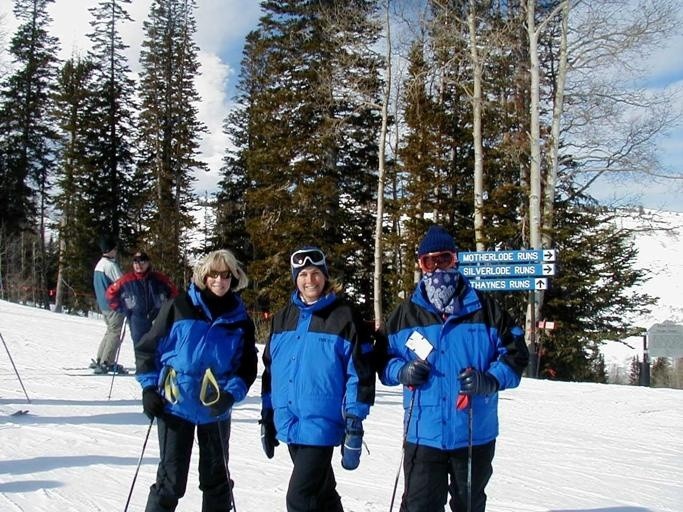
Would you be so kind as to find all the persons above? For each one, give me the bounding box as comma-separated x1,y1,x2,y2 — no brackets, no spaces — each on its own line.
372,225,528,510
260,245,376,510
107,251,179,361
91,236,129,373
133,249,258,510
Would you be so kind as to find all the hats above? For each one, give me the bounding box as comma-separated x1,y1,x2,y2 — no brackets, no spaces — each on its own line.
131,250,150,261
98,236,117,254
417,223,455,255
291,246,330,286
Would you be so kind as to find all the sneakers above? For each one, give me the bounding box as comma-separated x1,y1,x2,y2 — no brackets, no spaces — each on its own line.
92,359,102,367
98,363,124,373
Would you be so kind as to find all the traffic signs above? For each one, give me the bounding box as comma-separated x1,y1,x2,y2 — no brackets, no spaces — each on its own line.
464,277,549,291
457,263,555,278
457,248,556,263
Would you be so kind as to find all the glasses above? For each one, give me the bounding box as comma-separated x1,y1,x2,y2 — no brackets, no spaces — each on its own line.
206,270,233,279
420,250,455,273
133,261,149,265
291,249,326,268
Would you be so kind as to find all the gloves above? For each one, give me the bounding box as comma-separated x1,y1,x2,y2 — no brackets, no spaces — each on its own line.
143,385,166,419
398,357,433,387
117,306,132,319
203,391,235,416
456,367,498,395
259,410,280,459
338,430,363,471
